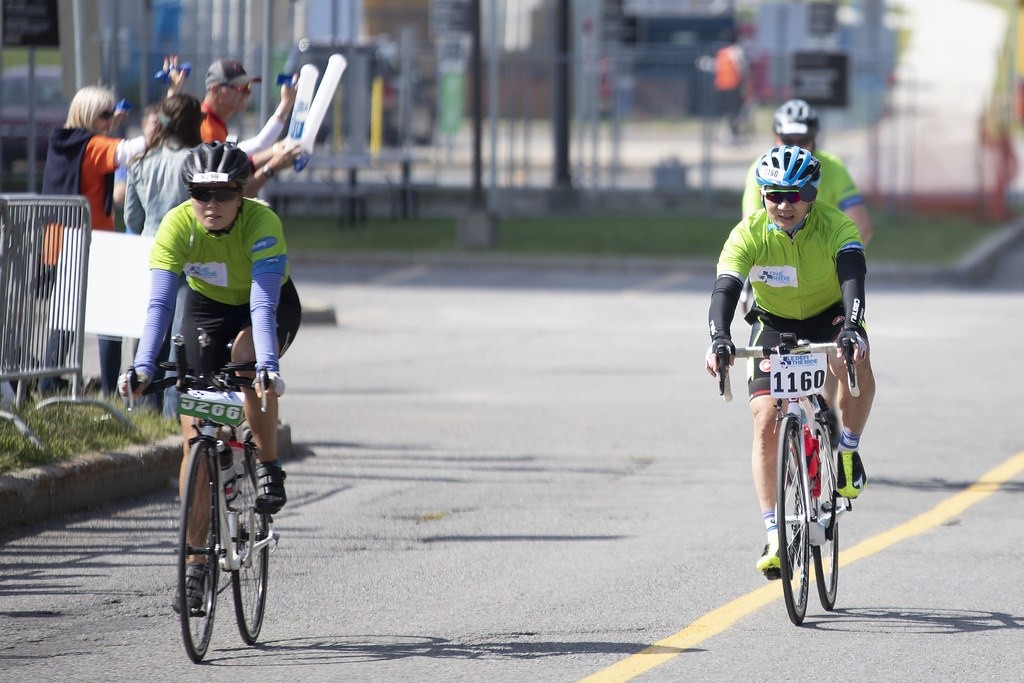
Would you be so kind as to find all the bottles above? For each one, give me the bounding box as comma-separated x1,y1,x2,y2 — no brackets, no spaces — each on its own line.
790,425,822,498
228,436,245,509
217,439,242,502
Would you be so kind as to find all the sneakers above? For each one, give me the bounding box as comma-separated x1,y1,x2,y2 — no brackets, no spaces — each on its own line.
254,467,288,514
836,447,869,498
173,563,210,613
757,542,792,579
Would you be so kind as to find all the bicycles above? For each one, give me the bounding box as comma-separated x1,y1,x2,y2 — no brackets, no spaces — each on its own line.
714,330,848,626
121,335,275,665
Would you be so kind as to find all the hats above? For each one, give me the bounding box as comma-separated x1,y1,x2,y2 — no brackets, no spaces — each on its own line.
205,57,262,90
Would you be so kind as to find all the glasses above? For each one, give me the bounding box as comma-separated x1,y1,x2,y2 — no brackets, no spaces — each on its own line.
760,187,801,204
100,111,114,120
776,132,814,148
221,82,252,96
187,185,243,202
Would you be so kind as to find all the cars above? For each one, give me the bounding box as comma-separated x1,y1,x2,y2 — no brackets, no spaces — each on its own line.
0,70,68,172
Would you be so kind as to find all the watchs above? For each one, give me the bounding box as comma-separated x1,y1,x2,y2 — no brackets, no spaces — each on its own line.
262,162,273,178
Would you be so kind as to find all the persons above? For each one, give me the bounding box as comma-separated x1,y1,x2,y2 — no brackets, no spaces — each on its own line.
230,136,301,198
35,55,186,402
122,93,216,412
741,99,874,250
116,142,303,616
586,54,616,121
714,22,769,147
199,59,299,155
705,146,875,580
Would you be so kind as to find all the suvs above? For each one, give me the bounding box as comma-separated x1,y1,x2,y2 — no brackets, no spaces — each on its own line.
281,42,407,149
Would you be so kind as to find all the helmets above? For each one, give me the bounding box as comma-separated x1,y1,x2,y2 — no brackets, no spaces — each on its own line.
181,140,250,189
773,99,821,136
756,146,822,191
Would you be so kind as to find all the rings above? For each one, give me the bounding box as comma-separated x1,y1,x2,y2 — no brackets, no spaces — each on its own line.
169,64,175,70
